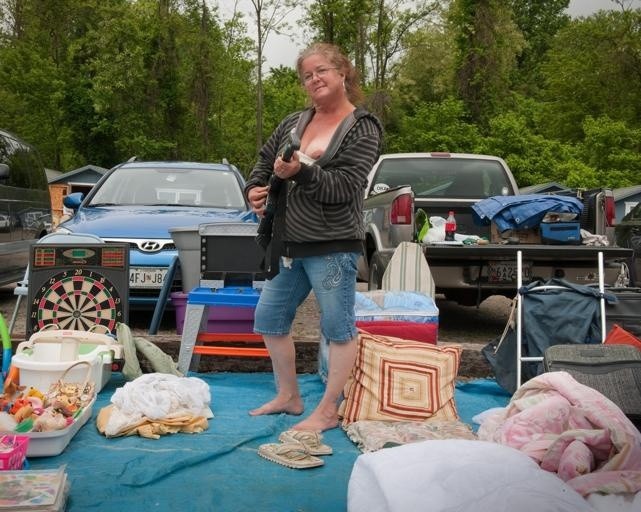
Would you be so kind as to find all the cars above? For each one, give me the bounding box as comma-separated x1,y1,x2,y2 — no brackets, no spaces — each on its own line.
30,156,261,306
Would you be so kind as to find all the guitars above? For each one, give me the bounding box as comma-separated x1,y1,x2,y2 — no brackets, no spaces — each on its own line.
255,127,301,251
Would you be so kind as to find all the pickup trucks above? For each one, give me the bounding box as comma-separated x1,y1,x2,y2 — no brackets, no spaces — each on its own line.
357,153,633,305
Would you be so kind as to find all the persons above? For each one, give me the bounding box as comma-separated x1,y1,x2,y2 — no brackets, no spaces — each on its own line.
243,43,383,433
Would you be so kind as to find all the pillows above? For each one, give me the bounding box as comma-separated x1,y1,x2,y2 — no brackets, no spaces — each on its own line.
334,325,480,455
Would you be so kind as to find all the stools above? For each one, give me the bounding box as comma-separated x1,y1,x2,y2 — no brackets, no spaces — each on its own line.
176,285,270,374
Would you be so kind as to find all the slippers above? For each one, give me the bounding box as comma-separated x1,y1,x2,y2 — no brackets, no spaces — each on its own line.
257,430,332,469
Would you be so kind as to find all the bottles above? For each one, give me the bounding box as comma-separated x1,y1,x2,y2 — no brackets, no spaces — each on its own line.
445,211,457,241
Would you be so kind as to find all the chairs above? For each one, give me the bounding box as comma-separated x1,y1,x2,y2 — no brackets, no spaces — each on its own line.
134,183,228,206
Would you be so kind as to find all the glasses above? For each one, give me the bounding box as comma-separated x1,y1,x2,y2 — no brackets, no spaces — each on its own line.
303,65,339,82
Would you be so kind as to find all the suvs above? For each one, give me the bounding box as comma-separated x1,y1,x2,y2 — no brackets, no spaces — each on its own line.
0,127,55,287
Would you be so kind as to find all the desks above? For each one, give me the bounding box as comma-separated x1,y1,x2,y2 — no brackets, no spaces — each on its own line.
411,245,634,394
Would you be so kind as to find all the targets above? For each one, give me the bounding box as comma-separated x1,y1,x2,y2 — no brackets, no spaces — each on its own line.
30,270,122,336
